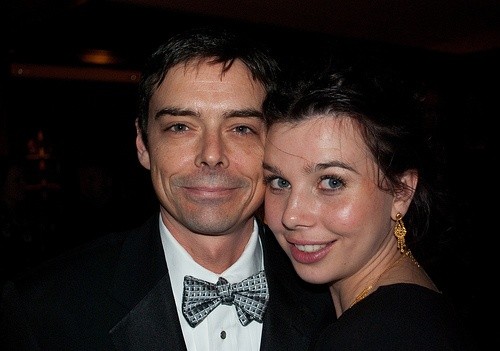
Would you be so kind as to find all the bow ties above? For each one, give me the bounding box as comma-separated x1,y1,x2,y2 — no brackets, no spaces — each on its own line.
180,270,270,328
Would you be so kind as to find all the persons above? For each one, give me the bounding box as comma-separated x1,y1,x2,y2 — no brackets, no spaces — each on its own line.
257,62,475,351
19,24,338,351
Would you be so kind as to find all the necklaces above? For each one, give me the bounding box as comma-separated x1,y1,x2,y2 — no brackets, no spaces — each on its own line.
346,249,413,312
